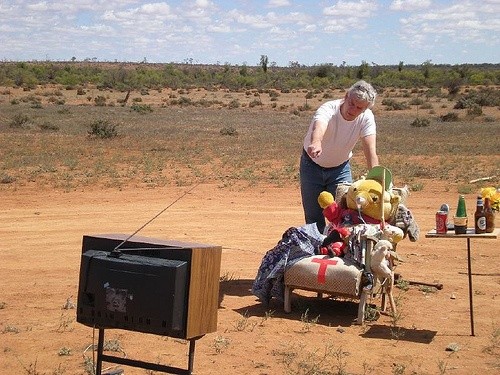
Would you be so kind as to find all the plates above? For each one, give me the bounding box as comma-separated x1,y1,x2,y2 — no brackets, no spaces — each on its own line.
447,223,453,229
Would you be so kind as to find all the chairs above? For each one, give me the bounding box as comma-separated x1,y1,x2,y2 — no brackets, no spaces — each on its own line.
280,183,406,328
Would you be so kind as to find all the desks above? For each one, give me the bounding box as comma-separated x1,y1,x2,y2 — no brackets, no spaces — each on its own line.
426,224,500,336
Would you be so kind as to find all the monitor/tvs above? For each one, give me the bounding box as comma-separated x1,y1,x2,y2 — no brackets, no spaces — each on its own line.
76,234,222,338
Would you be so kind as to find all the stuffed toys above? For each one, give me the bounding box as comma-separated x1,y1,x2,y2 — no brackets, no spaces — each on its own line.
314,164,402,258
370,239,393,293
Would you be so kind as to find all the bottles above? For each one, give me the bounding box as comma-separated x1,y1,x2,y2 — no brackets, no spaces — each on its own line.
454,194,467,234
482,197,494,234
475,194,487,234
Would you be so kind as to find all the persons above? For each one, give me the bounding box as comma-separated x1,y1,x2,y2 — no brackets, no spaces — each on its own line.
300,80,378,235
